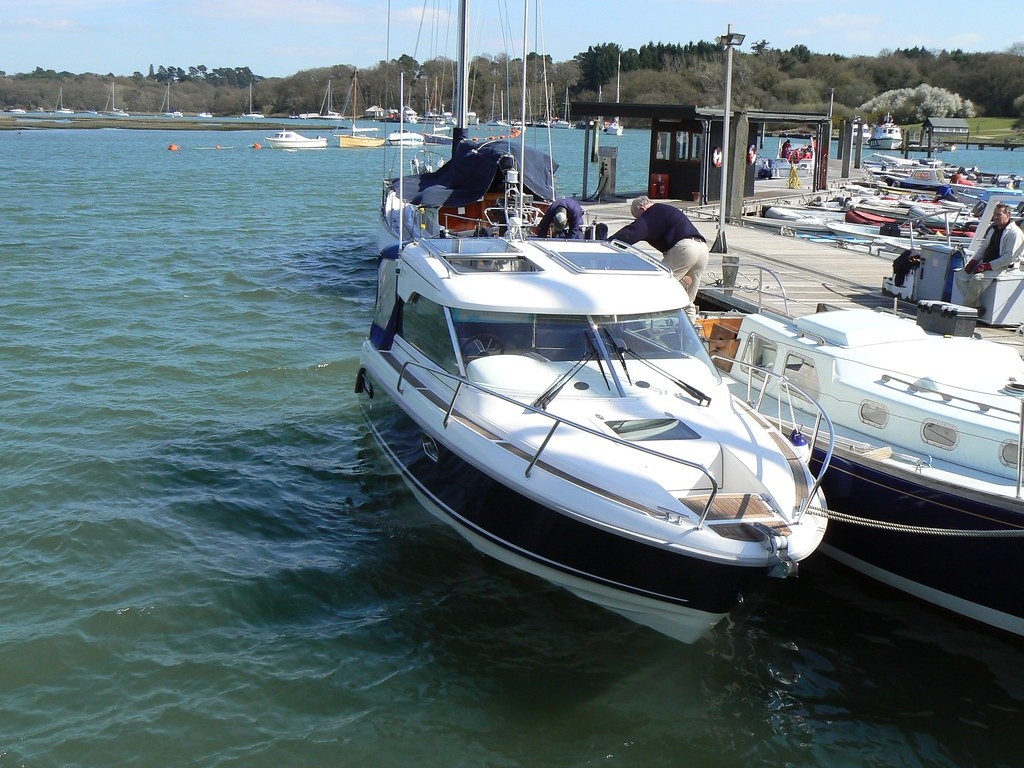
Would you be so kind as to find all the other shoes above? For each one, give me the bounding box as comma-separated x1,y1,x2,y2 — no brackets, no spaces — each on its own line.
972,306,986,317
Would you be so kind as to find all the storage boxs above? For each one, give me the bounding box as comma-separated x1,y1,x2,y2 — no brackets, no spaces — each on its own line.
916,300,980,339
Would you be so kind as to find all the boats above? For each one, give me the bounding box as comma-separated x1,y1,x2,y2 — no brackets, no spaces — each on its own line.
867,112,904,151
196,112,213,118
674,254,1023,533
906,138,954,152
851,113,872,146
265,128,329,148
387,126,426,147
760,149,1023,268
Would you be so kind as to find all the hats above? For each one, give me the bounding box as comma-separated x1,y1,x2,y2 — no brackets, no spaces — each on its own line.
553,207,567,228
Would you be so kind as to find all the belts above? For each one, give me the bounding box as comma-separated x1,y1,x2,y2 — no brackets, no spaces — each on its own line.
685,236,705,242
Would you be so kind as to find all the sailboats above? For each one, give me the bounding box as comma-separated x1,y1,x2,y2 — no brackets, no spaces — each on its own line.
421,70,455,145
351,1,837,646
52,84,75,114
235,82,265,120
374,53,624,136
95,80,130,117
293,78,345,121
330,65,387,147
155,80,185,119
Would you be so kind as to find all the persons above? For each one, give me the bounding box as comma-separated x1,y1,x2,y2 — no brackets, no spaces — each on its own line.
955,204,1024,317
781,139,792,157
534,199,586,240
610,195,710,302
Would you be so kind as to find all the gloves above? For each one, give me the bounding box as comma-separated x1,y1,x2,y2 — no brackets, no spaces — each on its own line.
965,259,979,274
974,262,991,274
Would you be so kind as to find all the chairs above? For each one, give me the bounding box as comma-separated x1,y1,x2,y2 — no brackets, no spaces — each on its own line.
583,338,628,359
452,336,486,357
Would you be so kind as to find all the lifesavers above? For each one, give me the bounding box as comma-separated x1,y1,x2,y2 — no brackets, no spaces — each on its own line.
748,145,757,165
713,148,723,167
